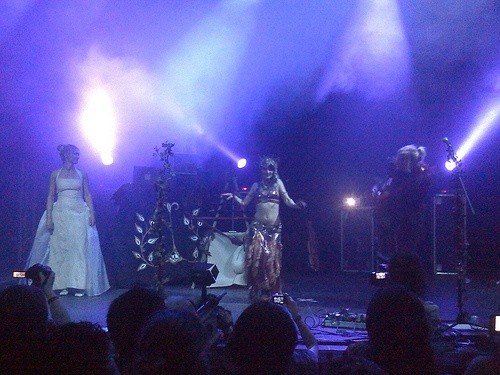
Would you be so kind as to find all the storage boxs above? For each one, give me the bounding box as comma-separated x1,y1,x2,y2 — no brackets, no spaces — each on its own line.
433,194,466,275
342,208,377,273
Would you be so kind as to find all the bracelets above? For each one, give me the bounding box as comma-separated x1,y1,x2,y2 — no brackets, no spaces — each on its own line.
293,311,299,317
233,193,234,199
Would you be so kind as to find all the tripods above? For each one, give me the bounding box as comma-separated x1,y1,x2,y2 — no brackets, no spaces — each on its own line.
432,172,489,336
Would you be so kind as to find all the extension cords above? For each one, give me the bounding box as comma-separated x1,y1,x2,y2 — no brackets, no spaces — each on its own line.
324,319,367,330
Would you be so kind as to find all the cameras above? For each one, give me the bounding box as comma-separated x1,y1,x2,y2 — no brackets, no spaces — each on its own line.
489,313,500,335
12,263,51,281
371,271,390,284
272,292,286,305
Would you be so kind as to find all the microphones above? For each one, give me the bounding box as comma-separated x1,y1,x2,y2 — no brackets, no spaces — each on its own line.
442,137,453,151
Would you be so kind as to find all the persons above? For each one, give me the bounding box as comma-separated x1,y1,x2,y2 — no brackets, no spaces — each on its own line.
0,255,500,375
22,144,110,297
220,158,306,304
375,145,433,270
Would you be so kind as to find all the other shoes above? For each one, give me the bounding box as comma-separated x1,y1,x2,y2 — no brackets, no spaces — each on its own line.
60,289,69,295
75,290,85,296
